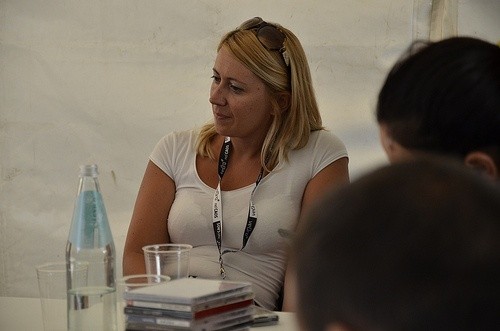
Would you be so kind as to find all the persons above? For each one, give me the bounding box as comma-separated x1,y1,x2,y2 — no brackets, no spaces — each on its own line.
282,155,500,331
120,15,354,315
373,32,500,177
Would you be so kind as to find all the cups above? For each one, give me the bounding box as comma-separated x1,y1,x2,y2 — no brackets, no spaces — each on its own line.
37,260,91,331
118,271,170,298
142,243,193,287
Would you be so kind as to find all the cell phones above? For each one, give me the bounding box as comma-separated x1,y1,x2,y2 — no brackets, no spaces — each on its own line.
250,304,278,328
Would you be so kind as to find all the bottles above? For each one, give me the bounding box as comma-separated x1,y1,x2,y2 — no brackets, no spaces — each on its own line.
64,163,121,330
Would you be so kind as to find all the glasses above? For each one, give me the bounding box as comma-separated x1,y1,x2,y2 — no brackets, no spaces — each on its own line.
242,17,290,71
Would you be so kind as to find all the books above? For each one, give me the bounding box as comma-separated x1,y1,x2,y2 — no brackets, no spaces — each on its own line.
122,275,258,331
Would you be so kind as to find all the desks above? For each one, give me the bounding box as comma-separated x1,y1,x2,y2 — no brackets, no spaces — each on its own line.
0,296,309,331
